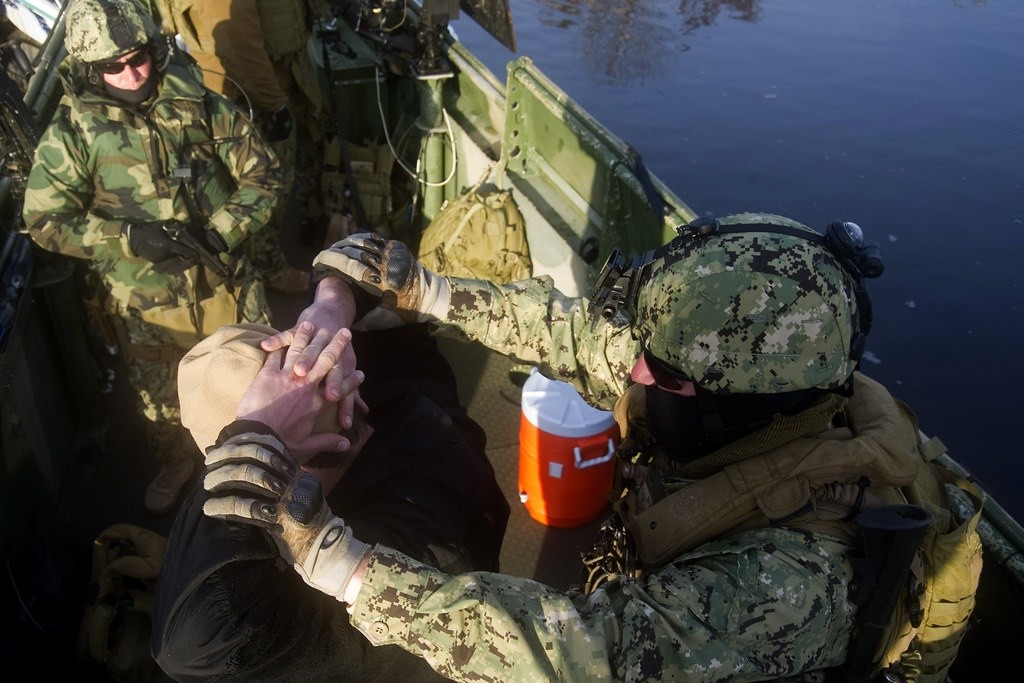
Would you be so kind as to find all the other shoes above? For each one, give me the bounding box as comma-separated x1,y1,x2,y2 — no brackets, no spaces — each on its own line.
269,266,311,295
146,460,194,513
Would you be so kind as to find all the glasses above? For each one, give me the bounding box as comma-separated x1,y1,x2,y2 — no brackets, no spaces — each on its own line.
94,46,152,75
640,344,691,391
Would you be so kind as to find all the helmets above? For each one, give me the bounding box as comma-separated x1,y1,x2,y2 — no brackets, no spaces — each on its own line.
64,0,157,61
629,210,863,395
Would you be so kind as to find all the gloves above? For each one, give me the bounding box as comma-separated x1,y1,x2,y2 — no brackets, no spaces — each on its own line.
312,233,447,334
266,111,293,142
202,432,372,601
128,220,228,273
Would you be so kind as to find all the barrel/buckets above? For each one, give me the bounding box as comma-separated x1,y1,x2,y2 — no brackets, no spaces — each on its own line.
518,365,619,528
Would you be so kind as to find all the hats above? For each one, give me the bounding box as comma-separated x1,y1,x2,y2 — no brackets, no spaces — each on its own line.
178,321,344,466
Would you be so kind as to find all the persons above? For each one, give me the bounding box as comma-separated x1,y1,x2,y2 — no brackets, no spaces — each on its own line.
0,0,885,682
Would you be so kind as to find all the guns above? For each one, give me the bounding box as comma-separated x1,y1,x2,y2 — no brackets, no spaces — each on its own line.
165,220,228,278
